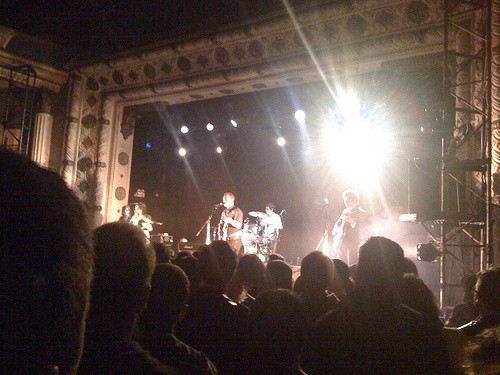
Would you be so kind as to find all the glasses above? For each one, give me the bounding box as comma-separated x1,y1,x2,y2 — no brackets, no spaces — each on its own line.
470,285,490,298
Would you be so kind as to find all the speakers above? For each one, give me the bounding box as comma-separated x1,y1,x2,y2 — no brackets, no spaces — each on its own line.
409,155,466,215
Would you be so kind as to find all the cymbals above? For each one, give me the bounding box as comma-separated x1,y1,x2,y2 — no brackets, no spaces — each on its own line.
248,211,267,218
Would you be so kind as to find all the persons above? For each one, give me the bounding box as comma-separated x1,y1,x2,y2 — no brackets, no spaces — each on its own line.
0,192,500,375
0,146,92,375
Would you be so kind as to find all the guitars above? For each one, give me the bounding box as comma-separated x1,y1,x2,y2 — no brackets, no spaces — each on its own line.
218,206,237,241
333,207,360,250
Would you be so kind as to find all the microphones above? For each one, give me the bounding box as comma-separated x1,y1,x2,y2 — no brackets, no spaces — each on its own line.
215,203,222,209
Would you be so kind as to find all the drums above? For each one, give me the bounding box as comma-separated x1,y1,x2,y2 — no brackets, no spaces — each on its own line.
253,237,271,254
